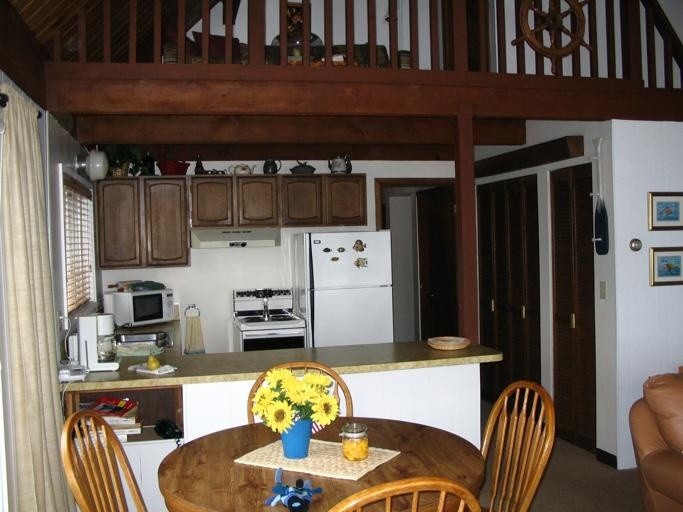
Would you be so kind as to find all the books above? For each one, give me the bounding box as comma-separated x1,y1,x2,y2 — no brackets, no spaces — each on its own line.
73,396,142,449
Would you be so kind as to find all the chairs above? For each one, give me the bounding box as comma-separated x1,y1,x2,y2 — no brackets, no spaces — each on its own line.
247,362,352,424
61,409,148,512
328,477,482,512
482,380,556,512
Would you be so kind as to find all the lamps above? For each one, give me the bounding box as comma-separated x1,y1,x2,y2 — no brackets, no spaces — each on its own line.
75,150,109,181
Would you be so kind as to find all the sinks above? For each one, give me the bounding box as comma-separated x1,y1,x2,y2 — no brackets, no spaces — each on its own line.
115,329,174,348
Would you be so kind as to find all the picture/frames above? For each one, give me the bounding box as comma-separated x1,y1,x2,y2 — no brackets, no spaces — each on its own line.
647,191,683,230
649,246,683,286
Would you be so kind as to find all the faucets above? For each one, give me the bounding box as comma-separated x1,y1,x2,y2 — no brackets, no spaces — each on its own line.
117,321,135,332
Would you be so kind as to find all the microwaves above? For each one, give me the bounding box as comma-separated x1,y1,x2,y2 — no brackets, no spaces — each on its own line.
105,289,175,328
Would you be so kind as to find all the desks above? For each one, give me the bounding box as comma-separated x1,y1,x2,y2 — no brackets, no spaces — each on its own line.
158,417,486,512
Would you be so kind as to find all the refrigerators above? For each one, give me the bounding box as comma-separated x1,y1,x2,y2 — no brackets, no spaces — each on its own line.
291,230,394,349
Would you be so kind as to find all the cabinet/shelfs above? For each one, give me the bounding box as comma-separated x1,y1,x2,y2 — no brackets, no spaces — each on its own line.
191,174,279,228
280,173,367,227
97,178,187,267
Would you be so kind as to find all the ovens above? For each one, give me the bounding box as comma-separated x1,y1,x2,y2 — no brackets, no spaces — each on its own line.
242,331,308,350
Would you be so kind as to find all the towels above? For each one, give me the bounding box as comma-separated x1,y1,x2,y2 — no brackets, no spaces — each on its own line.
182,317,205,357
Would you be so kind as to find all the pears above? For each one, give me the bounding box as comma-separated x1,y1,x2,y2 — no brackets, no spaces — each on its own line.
145,356,161,371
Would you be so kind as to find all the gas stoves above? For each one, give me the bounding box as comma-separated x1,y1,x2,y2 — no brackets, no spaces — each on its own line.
233,308,306,331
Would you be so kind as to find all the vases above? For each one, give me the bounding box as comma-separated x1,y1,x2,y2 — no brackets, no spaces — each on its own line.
281,420,312,459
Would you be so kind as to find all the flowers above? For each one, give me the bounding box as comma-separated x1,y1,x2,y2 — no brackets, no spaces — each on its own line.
251,367,341,434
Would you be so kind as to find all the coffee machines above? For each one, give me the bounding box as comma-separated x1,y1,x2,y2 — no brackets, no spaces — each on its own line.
69,315,121,371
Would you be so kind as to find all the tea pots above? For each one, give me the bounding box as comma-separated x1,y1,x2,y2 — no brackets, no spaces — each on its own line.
263,157,282,174
229,163,256,175
328,153,352,174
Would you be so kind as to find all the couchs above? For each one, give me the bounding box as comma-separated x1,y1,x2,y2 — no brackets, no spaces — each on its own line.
629,366,683,512
